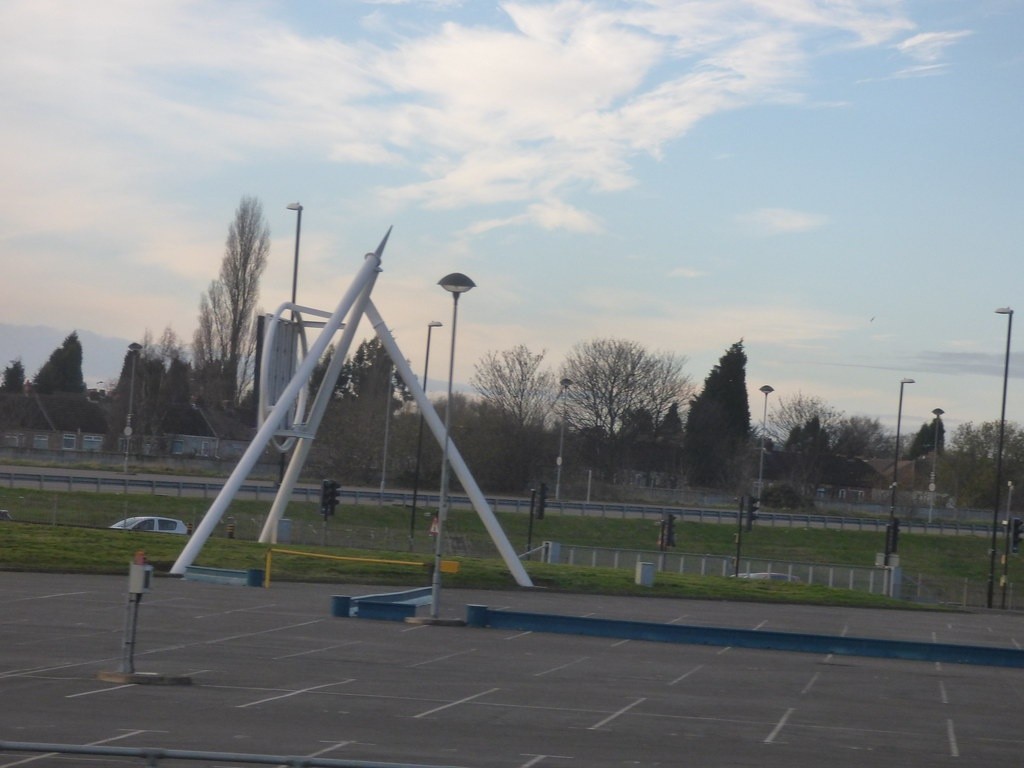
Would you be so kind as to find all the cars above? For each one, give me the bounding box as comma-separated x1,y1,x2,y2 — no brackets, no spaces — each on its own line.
110,516,188,535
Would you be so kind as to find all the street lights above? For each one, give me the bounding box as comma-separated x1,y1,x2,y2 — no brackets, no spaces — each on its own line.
124,340,143,475
986,305,1015,609
753,384,775,519
407,321,443,539
883,378,917,562
427,272,476,616
286,201,305,321
926,408,945,523
554,378,574,498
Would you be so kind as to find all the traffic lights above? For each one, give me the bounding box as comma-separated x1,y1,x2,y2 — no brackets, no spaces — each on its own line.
747,496,759,533
322,480,333,514
1009,516,1023,553
331,480,343,507
534,483,548,521
664,514,677,547
889,518,901,552
